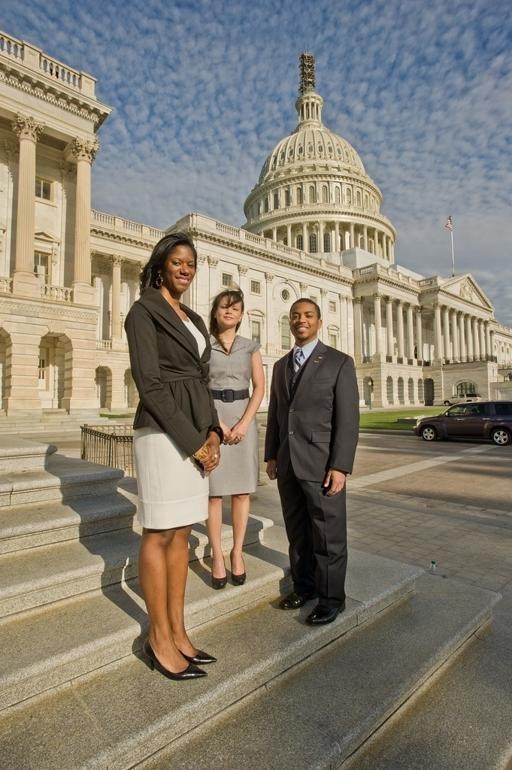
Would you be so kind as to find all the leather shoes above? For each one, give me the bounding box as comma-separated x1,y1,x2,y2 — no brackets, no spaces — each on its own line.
230,552,246,586
280,592,320,610
307,603,345,626
211,556,227,589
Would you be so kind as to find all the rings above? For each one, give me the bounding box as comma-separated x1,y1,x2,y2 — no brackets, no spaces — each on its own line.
214,454,218,458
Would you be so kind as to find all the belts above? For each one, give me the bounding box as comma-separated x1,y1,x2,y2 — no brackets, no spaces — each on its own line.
212,389,249,402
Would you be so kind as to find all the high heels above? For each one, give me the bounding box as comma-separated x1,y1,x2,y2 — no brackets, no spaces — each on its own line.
143,638,208,680
177,648,216,665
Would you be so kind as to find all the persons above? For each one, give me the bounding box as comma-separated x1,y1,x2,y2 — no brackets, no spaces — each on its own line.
123,230,224,680
198,288,265,589
265,297,360,625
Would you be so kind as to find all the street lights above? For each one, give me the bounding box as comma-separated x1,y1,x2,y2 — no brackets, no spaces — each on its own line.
367,380,373,412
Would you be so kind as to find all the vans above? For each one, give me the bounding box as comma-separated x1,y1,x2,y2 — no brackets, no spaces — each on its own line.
414,401,512,447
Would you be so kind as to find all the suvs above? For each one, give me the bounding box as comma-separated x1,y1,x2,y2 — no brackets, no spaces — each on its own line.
442,392,481,406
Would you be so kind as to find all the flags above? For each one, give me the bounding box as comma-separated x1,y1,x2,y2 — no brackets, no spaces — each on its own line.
446,216,453,231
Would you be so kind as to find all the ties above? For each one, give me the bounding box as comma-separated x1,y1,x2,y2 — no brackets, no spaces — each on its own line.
294,349,303,373
